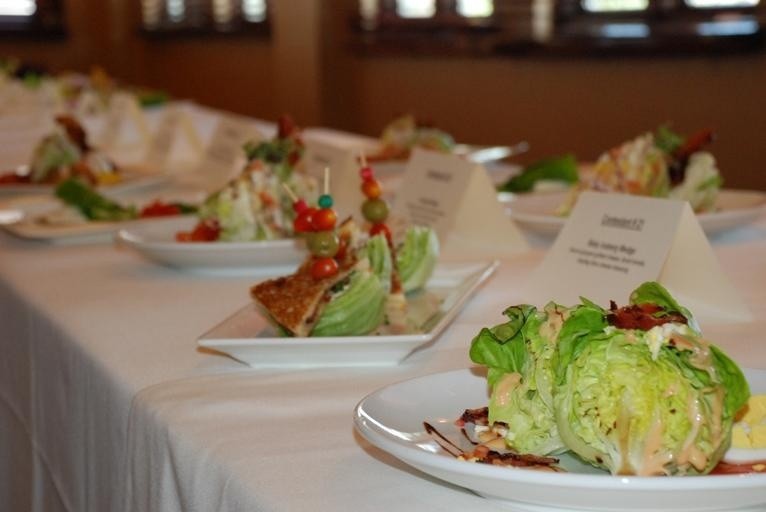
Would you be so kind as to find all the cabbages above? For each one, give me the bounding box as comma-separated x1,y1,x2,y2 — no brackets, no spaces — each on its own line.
274,227,439,337
469,282,751,474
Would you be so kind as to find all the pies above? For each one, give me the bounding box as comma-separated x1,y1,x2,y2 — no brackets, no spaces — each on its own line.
249,247,361,337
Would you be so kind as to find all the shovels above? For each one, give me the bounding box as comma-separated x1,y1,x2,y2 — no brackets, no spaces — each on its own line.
249,247,361,337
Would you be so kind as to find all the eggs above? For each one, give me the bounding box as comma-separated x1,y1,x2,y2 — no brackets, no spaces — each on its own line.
274,227,439,337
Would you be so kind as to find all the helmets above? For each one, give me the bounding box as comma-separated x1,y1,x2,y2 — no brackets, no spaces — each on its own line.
119,216,306,274
0,202,119,245
355,365,765,511
0,169,175,197
498,190,765,239
195,258,499,370
